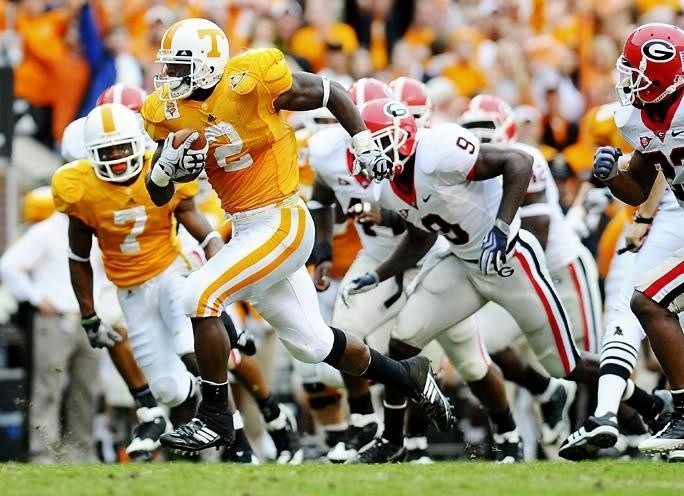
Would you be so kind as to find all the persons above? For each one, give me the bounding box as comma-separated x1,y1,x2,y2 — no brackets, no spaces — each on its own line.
51,103,253,463
0,214,100,464
141,18,456,457
293,105,340,197
293,78,522,463
592,24,684,453
389,72,432,127
341,99,676,463
565,101,641,307
457,95,614,443
536,88,580,218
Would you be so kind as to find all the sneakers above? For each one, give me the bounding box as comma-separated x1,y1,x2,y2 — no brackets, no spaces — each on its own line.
636,406,684,455
221,426,259,469
531,379,579,463
235,329,256,358
327,427,384,469
342,432,409,468
493,427,524,464
644,388,672,431
400,352,458,439
158,379,235,458
269,421,304,466
557,411,621,462
123,403,175,463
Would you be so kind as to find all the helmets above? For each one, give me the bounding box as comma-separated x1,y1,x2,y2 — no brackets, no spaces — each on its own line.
82,101,153,187
457,92,517,139
358,98,420,181
613,21,684,111
386,76,432,129
153,17,231,103
345,75,390,106
94,77,149,118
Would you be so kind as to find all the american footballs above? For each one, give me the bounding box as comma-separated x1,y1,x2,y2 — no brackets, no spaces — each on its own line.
172,127,207,183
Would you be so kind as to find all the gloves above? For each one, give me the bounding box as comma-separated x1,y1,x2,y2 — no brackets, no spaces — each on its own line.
476,217,517,280
347,129,394,186
149,129,210,198
338,268,381,309
588,143,621,182
77,310,126,355
